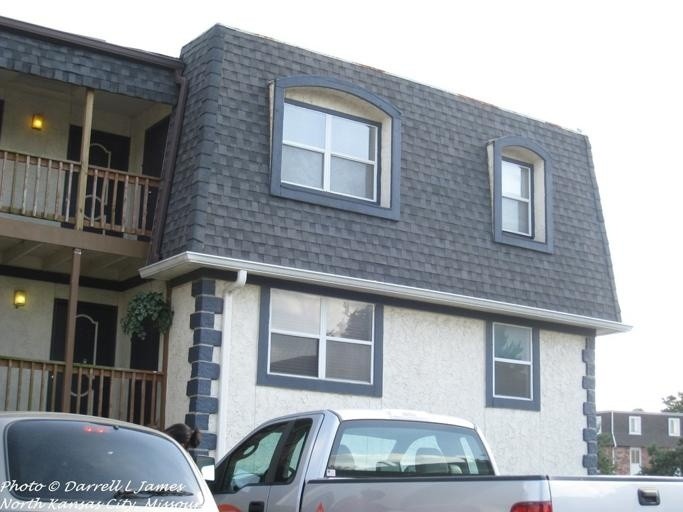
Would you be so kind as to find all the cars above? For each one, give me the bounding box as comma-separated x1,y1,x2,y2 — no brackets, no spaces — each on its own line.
1,411,217,512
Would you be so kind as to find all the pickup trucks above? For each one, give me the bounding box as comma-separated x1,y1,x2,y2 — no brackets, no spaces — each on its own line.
213,410,682,512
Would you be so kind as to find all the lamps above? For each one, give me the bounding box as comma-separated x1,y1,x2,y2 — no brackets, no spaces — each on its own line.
14,289,27,308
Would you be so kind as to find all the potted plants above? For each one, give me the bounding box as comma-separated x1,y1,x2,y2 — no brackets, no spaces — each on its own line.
120,291,174,344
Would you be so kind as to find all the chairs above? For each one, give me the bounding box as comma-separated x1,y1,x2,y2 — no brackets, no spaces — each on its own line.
332,445,449,476
